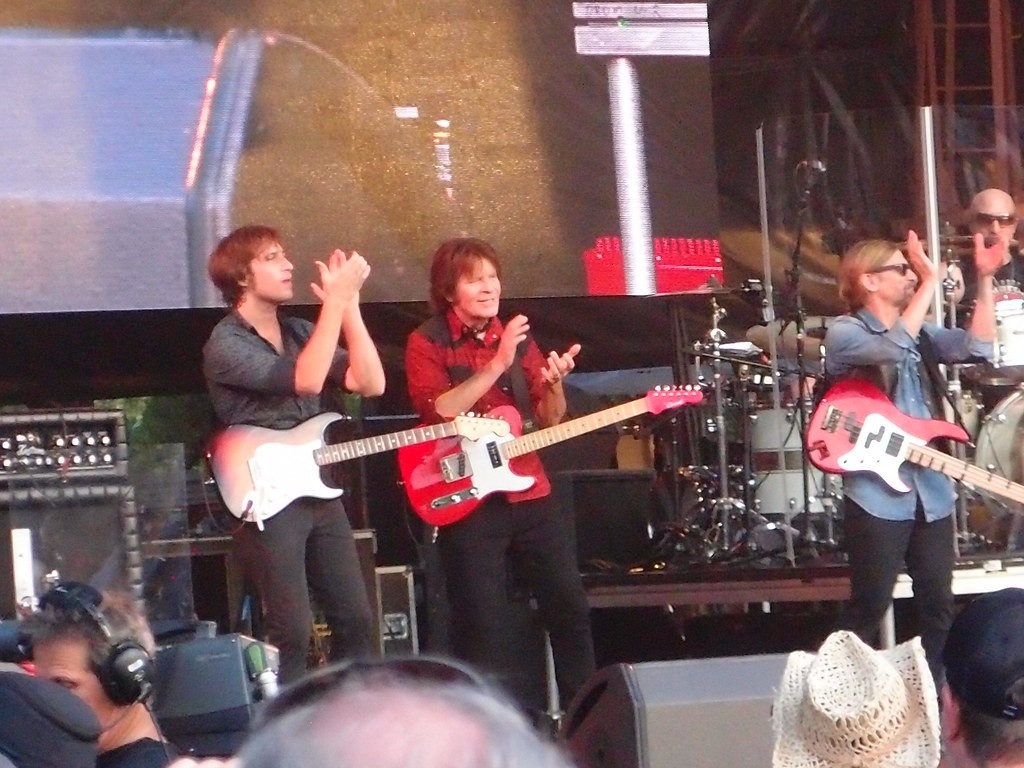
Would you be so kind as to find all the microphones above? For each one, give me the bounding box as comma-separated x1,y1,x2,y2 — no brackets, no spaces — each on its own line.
800,160,822,172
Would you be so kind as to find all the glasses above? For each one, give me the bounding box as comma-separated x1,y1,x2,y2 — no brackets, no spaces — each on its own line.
970,211,1017,227
864,262,914,276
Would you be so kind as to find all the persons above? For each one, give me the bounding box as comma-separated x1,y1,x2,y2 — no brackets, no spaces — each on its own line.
19,579,182,768
822,231,1011,696
200,225,385,690
406,238,596,721
937,188,1024,332
236,660,578,768
941,588,1024,768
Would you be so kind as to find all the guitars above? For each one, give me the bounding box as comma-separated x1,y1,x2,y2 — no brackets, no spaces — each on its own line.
397,384,703,528
208,410,510,522
805,378,1024,504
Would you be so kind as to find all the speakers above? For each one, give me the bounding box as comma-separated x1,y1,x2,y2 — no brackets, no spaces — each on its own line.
0,486,147,625
554,467,663,582
147,633,257,760
556,652,790,767
300,526,385,668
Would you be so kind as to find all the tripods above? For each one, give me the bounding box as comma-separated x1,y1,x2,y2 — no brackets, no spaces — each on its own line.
640,171,842,573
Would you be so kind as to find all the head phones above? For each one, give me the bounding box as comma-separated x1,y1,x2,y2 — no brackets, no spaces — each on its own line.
39,581,158,706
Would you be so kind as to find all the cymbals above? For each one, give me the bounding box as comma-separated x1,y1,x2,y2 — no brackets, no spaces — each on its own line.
894,234,1022,256
645,287,741,302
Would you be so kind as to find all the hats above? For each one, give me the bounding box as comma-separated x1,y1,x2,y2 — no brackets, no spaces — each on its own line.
770,629,942,768
942,588,1024,720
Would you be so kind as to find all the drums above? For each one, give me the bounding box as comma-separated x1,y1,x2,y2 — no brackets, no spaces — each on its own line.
946,390,986,460
748,399,839,523
962,297,1024,386
972,383,1024,517
